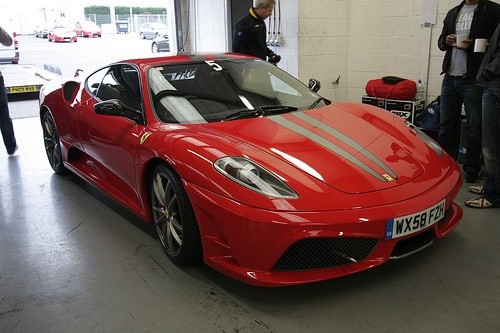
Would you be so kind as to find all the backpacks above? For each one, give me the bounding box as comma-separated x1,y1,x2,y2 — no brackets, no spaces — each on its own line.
415,95,441,130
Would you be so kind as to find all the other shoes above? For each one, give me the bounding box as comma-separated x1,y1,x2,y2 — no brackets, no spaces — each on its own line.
469,185,485,195
466,167,480,183
464,195,496,208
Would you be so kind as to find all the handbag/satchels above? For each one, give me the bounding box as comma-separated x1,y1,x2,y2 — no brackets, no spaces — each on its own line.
365,79,417,101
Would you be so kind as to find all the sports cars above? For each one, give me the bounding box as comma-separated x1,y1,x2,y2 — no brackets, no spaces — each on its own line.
37,50,465,289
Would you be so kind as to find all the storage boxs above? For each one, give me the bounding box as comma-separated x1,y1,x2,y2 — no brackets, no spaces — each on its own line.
362,96,425,125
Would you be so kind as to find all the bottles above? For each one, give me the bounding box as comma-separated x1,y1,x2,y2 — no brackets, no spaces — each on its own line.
414,79,424,100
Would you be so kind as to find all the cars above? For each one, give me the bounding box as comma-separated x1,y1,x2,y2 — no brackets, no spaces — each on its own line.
34,27,50,40
151,37,171,53
0,25,20,63
73,20,102,38
139,21,169,40
47,24,78,44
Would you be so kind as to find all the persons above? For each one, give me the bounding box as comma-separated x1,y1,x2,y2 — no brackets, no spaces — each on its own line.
437,0,500,184
233,0,281,97
464,24,500,209
0,26,18,155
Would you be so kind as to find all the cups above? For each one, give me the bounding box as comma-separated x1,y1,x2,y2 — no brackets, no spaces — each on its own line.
474,39,487,53
453,33,468,46
